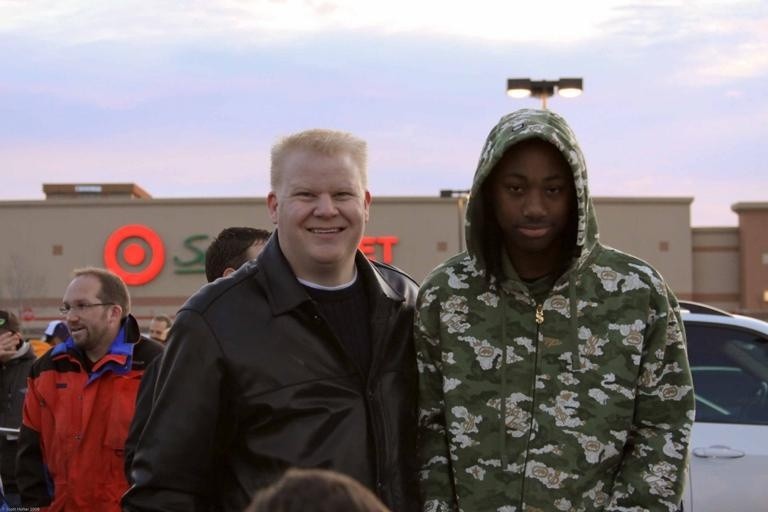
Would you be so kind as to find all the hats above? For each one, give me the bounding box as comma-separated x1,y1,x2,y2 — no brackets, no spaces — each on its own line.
0,311,20,333
44,320,72,337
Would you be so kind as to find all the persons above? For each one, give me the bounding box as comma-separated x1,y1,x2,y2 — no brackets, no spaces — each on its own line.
44,320,70,345
0,310,40,507
149,313,172,341
119,130,422,512
205,226,273,283
411,108,697,512
13,268,166,512
245,467,392,512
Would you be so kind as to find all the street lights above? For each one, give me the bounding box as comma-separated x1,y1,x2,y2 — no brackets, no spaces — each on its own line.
507,76,582,109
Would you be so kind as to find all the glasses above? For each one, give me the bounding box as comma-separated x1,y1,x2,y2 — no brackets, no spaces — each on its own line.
58,303,111,313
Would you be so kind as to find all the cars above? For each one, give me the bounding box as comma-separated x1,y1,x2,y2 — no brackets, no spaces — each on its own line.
662,296,766,510
